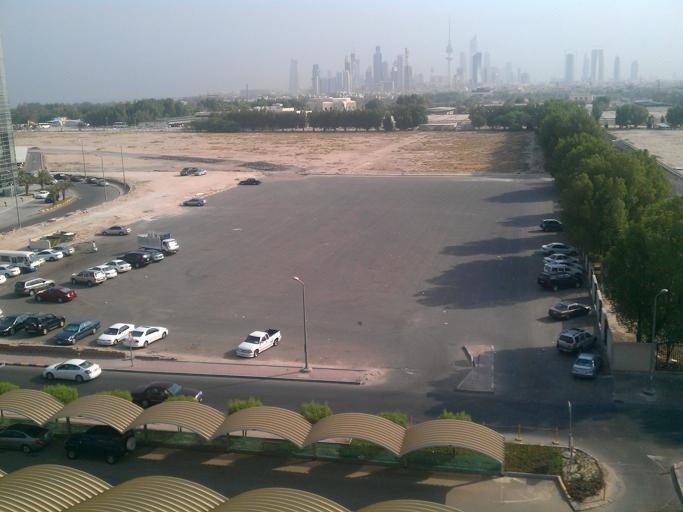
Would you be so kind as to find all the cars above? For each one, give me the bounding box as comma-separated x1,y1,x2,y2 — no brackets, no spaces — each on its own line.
18,121,53,130
0,224,164,303
53,172,110,187
112,121,129,130
537,242,601,291
42,358,102,383
34,189,57,203
180,167,208,176
556,328,602,379
539,218,566,232
0,423,54,454
548,300,592,320
166,122,184,127
239,177,261,185
0,312,168,349
183,196,206,207
131,380,204,408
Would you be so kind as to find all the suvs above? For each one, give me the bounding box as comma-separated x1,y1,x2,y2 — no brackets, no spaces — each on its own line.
62,424,137,465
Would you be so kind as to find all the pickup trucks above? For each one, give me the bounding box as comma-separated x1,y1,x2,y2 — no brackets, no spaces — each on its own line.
235,328,282,358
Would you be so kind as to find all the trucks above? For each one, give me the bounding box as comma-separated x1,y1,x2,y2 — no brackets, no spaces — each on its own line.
137,233,180,256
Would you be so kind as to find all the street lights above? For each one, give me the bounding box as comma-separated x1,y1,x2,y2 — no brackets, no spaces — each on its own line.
292,276,313,373
5,170,21,228
641,288,669,396
79,137,87,176
567,401,573,451
92,153,107,202
